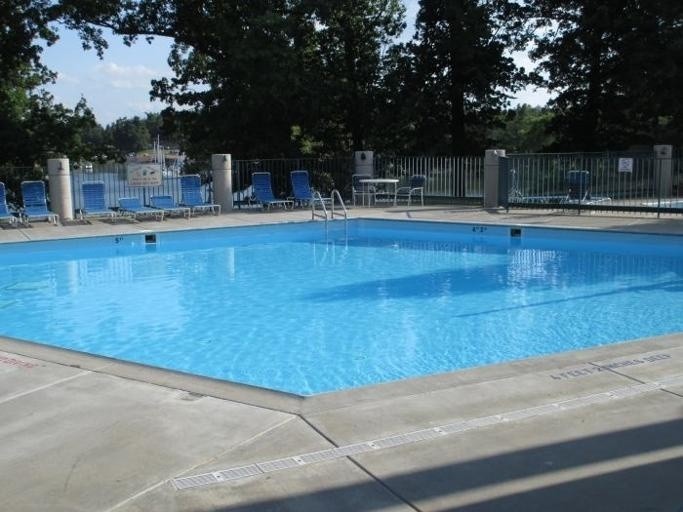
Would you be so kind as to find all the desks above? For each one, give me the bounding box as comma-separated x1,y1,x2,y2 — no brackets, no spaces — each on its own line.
359,178,399,208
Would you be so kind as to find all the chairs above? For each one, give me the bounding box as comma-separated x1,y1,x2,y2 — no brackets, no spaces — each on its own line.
352,173,376,208
0,170,332,230
395,175,426,207
560,169,613,217
